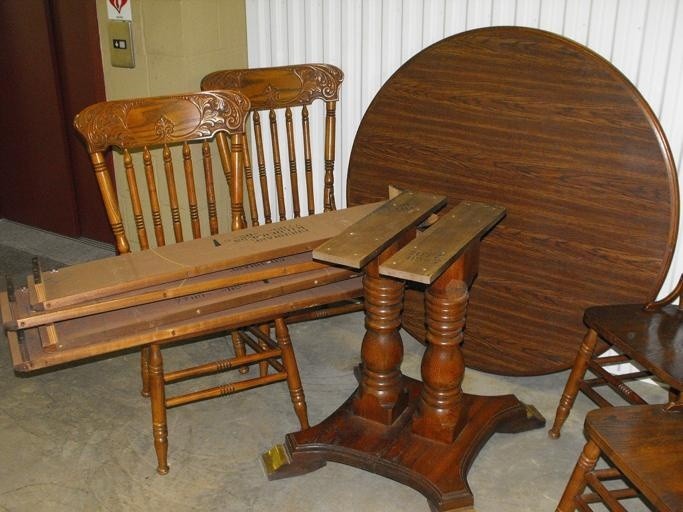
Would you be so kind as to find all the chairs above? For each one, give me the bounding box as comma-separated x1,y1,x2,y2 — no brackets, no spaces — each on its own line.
74,89,313,476
201,64,345,381
548,276,682,512
